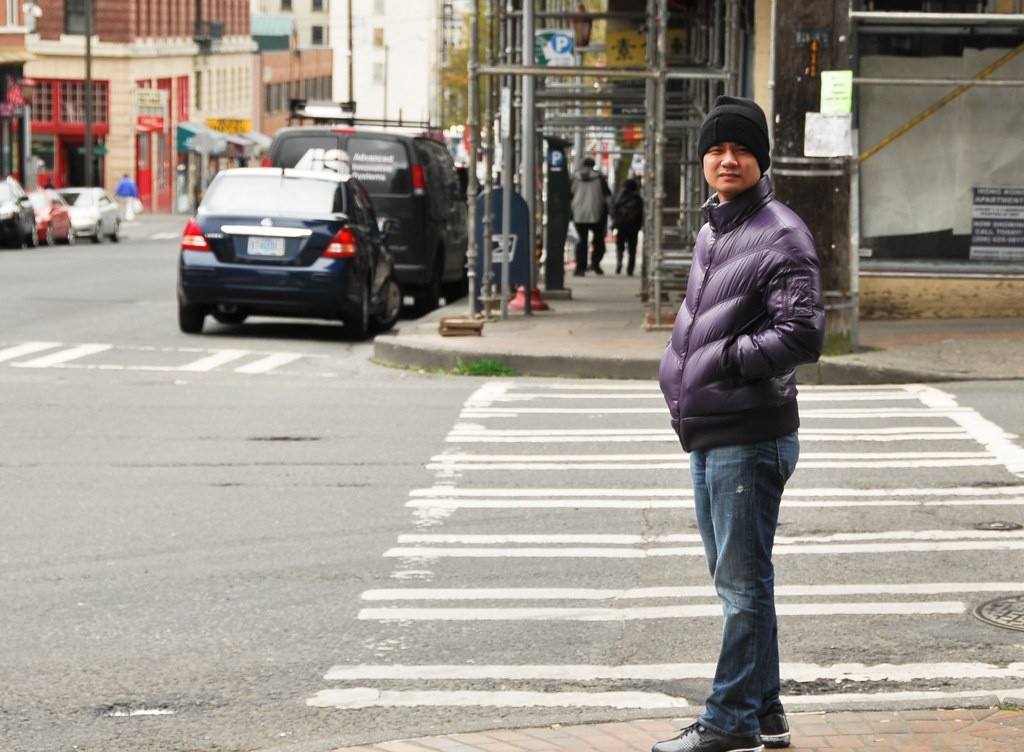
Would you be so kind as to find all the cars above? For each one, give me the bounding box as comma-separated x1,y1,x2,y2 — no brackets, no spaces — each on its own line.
174,164,404,334
26,192,77,246
0,176,40,249
55,185,122,244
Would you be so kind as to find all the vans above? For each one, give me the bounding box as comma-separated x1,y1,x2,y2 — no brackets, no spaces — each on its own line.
259,115,469,315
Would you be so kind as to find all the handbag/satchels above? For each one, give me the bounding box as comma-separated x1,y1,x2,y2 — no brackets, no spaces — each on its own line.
133,197,144,215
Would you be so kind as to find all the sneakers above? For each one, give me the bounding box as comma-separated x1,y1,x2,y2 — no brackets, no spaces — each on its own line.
652,719,765,752
759,704,790,749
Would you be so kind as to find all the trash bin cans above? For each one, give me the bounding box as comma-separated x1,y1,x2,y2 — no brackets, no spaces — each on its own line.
475,184,532,313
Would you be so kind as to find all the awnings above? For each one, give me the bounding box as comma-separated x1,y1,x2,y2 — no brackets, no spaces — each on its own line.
177,121,273,158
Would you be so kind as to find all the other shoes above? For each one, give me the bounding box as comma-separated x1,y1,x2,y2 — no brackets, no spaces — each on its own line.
591,263,603,275
573,267,584,275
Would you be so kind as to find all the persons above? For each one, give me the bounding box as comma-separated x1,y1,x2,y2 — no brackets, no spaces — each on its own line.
115,174,137,222
658,96,825,752
569,158,611,277
610,179,644,277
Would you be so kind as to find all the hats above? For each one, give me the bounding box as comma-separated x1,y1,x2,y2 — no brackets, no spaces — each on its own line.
697,94,770,176
